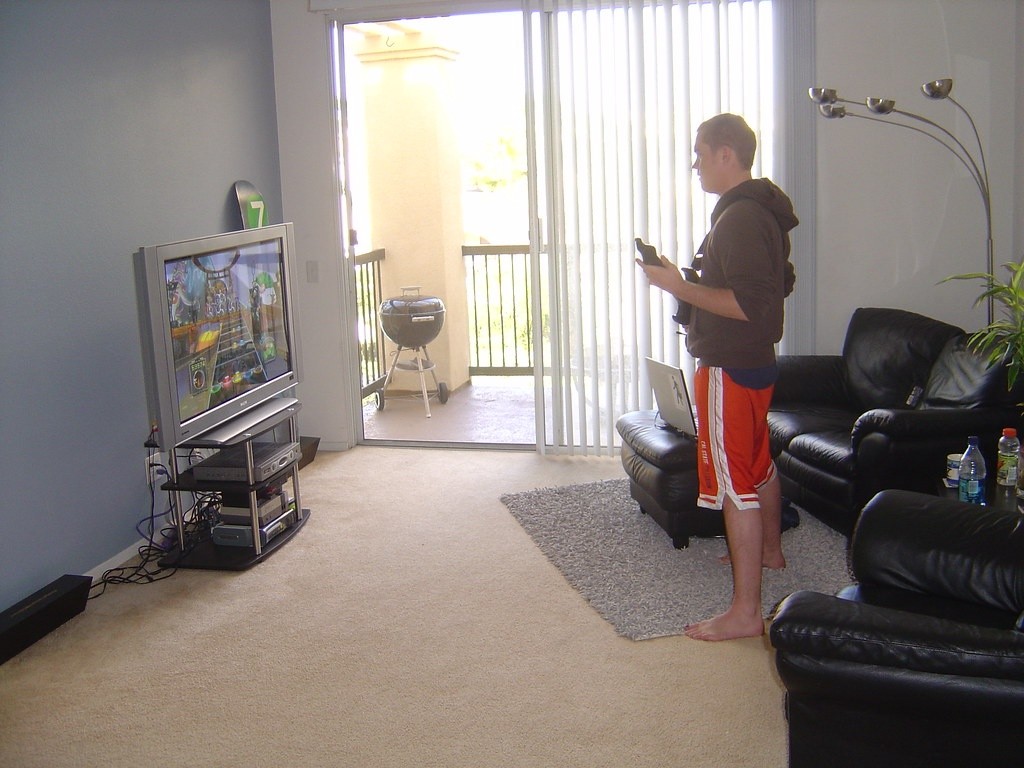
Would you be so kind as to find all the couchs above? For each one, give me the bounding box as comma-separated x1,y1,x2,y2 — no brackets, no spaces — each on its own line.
770,489,1024,768
768,307,1024,550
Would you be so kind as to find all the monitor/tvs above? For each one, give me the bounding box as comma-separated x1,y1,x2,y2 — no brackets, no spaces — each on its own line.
132,220,304,452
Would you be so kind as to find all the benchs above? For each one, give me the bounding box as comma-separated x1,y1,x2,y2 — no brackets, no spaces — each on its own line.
616,405,799,549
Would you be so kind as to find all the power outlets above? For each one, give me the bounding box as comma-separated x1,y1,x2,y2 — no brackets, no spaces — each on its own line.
146,452,163,484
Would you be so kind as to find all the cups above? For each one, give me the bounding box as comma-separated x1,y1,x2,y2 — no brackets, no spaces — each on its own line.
946,453,965,486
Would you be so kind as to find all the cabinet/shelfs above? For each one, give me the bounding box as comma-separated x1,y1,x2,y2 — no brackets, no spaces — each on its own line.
144,403,311,570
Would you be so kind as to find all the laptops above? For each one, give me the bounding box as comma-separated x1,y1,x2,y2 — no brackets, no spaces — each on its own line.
645,356,700,440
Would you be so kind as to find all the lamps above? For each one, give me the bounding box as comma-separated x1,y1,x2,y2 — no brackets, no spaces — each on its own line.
808,78,993,333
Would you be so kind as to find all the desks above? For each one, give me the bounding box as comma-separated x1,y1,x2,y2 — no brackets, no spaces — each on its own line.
933,477,1024,511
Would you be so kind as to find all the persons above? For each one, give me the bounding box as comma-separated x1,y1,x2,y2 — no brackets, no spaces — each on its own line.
634,114,800,642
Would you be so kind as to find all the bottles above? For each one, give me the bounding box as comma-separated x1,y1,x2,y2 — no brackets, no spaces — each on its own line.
996,428,1021,485
958,436,986,506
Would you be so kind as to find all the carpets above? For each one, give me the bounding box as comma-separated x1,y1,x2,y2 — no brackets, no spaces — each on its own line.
500,478,858,643
